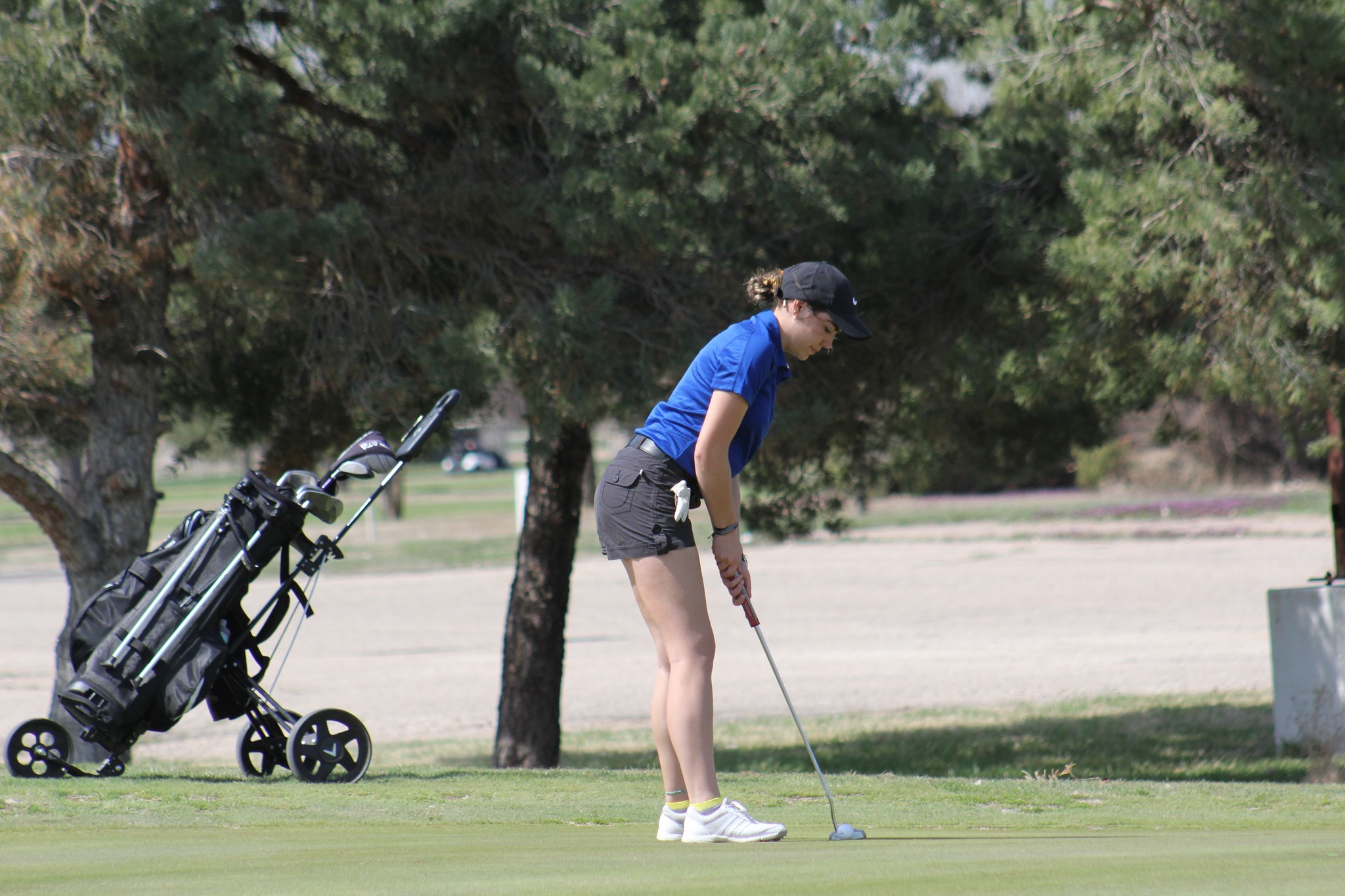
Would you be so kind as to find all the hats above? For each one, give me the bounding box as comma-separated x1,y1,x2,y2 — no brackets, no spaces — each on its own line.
776,260,872,341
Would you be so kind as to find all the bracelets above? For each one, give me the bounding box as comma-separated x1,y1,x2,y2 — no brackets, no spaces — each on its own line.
707,522,739,539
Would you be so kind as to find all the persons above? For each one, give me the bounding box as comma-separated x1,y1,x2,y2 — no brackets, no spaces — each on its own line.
595,259,872,846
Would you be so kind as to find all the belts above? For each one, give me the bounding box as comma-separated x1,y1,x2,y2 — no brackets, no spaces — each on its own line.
627,434,703,499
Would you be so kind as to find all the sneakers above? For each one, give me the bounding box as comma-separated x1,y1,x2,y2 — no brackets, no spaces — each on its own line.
656,804,687,841
681,798,788,842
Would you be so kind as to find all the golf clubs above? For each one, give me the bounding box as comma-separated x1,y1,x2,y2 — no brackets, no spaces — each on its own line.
730,572,867,844
275,460,376,525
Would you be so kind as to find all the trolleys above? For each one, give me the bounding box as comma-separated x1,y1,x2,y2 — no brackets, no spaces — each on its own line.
5,389,460,785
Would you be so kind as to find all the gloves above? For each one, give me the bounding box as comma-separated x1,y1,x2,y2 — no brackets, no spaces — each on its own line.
672,478,691,521
